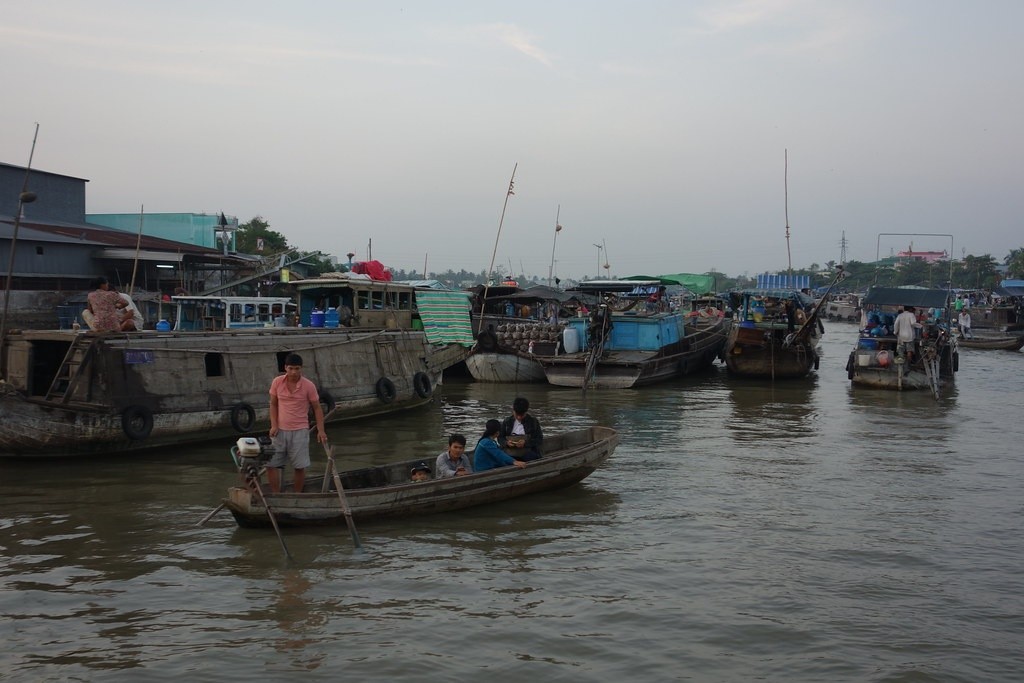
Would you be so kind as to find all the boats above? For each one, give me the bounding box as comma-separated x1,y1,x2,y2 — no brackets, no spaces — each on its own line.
465,341,559,382
949,326,1024,351
536,304,724,390
809,291,863,323
852,234,959,390
223,427,621,528
726,288,825,378
0,272,474,457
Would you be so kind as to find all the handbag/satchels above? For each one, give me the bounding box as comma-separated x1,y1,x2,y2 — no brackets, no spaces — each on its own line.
505,433,530,456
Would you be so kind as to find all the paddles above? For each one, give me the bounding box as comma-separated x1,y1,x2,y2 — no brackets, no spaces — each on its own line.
253,479,291,557
195,407,339,526
322,434,360,548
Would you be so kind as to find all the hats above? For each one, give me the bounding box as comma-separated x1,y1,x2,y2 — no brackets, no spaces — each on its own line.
409,460,432,475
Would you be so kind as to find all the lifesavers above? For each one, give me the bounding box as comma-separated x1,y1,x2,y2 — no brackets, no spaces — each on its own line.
376,377,396,404
679,358,689,376
121,404,154,440
308,391,335,419
703,349,713,365
414,372,431,398
231,403,256,434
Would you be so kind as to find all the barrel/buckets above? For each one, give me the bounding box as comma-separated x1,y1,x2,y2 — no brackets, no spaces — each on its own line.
412,318,422,330
155,319,170,331
506,304,514,315
858,354,870,366
754,306,764,316
310,307,340,328
563,326,579,354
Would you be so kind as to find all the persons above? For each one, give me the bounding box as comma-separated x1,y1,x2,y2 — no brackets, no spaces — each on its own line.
107,285,144,331
265,353,327,492
435,434,473,479
955,294,970,310
647,286,666,311
410,461,431,481
893,306,922,370
82,277,129,333
959,310,974,340
473,419,526,472
575,303,588,313
497,398,543,461
